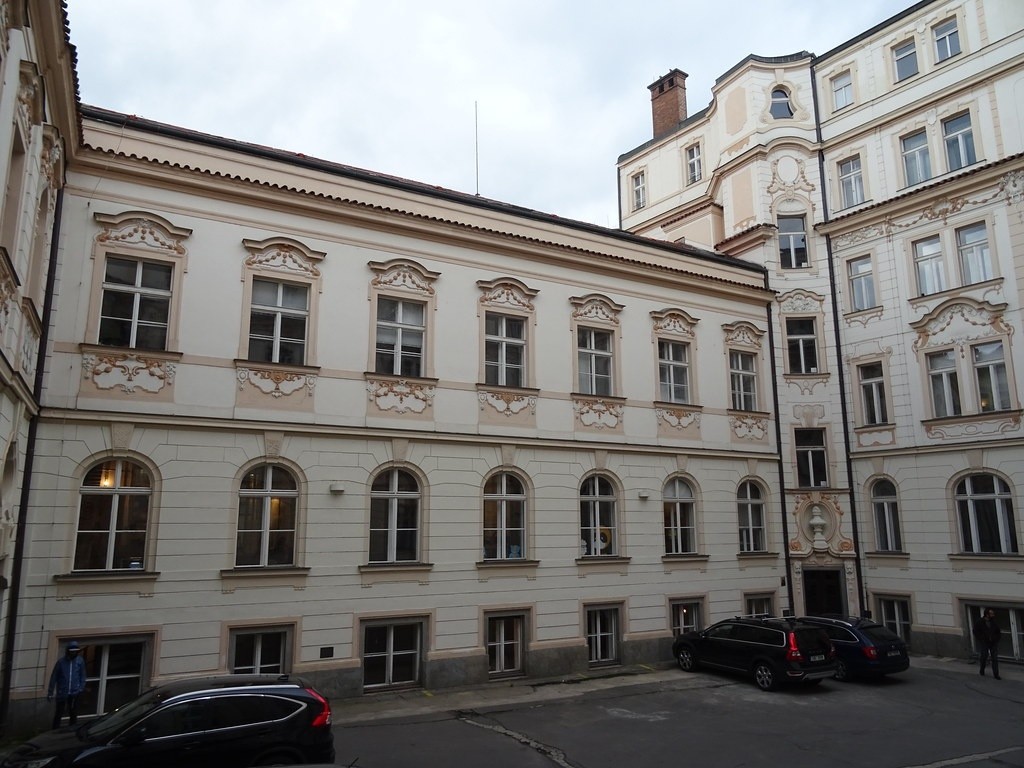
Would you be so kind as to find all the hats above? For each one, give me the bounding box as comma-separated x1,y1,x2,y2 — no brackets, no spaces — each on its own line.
66,641,80,651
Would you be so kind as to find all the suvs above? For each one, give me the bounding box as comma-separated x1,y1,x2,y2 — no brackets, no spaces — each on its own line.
780,615,910,681
3,674,336,768
672,616,836,691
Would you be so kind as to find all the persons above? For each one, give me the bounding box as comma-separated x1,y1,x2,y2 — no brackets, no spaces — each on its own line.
973,607,1002,680
46,641,86,730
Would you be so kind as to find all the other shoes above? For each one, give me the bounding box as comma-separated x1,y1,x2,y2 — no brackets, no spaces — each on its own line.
994,676,1001,680
980,668,984,675
